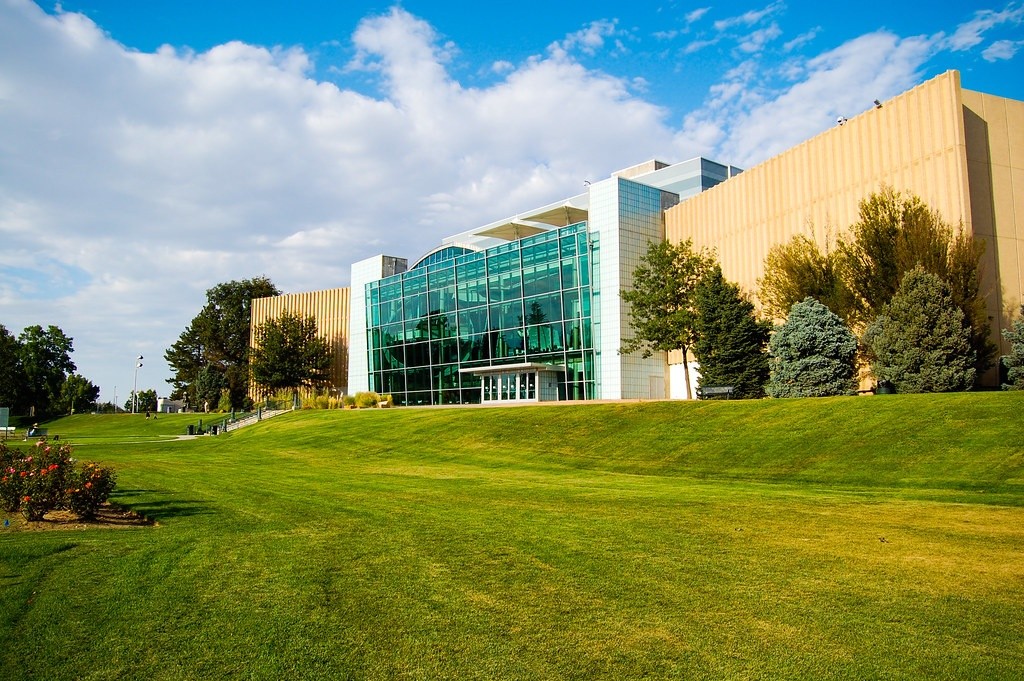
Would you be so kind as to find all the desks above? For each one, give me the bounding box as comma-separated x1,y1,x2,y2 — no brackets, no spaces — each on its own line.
0,426,16,438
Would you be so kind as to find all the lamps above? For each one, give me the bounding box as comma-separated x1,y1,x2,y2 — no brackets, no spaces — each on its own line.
873,99,882,109
837,116,847,126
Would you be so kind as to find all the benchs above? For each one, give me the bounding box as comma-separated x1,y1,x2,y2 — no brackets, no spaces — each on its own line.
21,428,49,440
697,385,735,401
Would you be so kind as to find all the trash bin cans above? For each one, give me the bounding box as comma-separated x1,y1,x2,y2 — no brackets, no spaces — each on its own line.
186,425,194,435
212,426,217,435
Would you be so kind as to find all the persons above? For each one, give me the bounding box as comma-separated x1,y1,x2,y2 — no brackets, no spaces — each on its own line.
204,400,209,414
22,423,39,442
146,411,158,420
